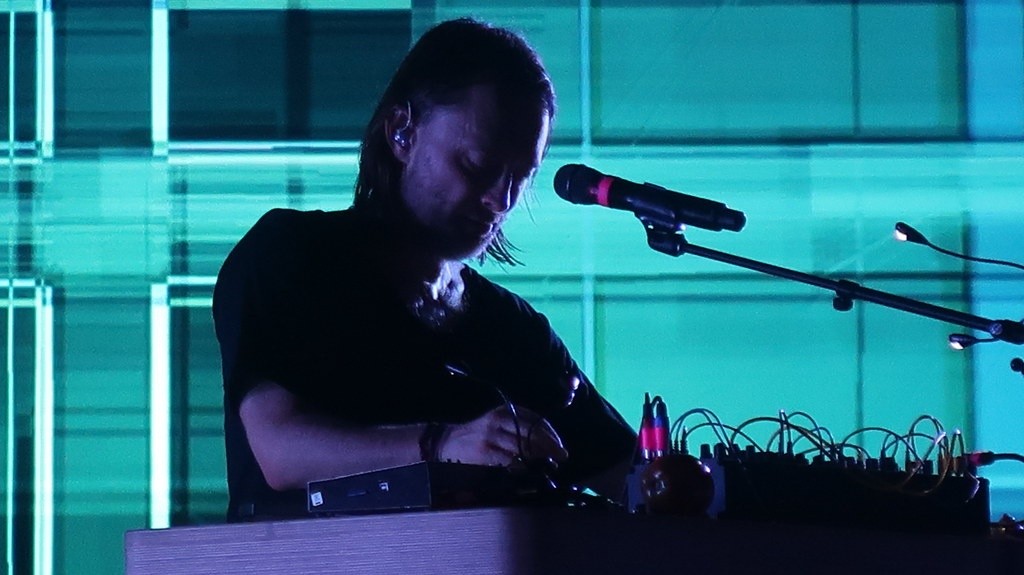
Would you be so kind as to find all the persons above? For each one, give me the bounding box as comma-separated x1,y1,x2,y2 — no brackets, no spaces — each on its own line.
213,18,640,522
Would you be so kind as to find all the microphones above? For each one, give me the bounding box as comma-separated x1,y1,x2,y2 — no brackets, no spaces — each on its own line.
554,164,746,232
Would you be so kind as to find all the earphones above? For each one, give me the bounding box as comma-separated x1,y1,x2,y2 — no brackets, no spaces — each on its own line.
396,127,412,151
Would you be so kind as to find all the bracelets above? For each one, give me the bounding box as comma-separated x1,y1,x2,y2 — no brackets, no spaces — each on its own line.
420,421,447,461
